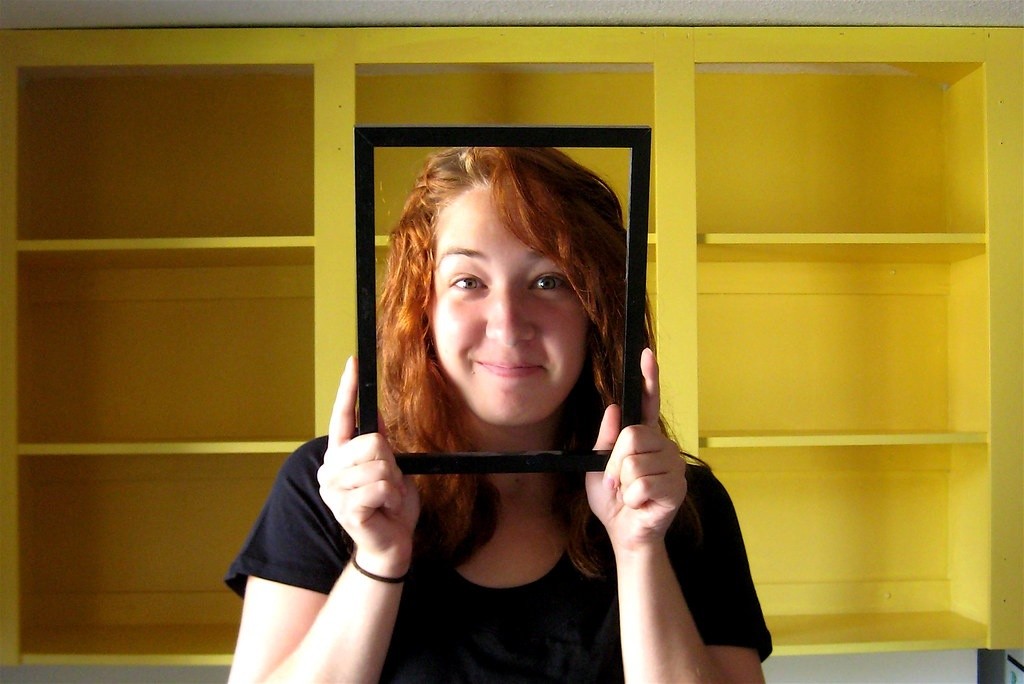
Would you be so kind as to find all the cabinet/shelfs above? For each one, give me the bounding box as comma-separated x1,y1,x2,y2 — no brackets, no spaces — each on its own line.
0,26,1024,666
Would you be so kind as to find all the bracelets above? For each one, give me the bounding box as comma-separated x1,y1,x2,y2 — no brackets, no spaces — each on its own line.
351,550,408,583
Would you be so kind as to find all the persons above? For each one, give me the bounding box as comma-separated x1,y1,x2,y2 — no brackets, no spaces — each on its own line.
224,146,773,684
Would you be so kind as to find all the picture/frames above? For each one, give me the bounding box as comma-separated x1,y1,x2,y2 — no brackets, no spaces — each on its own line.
352,125,653,474
